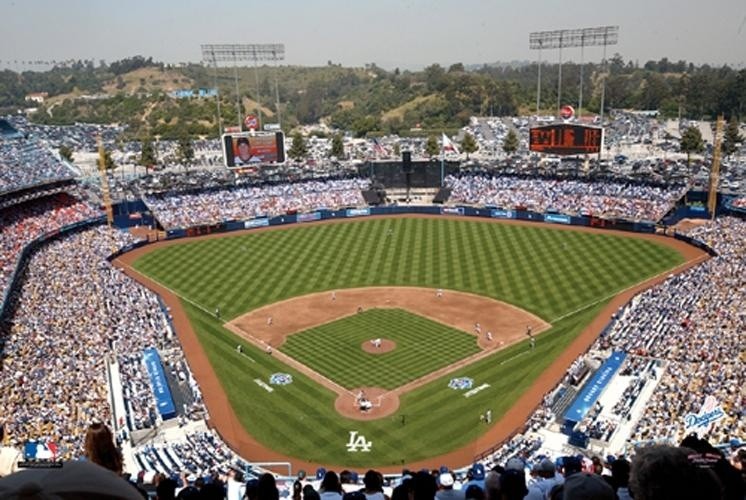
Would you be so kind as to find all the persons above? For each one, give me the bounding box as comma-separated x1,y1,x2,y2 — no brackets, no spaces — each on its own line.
1,176,376,500
233,138,262,163
376,171,745,500
1,132,74,176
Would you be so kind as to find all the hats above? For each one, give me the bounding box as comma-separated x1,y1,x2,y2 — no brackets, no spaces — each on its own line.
730,440,739,446
297,455,617,496
